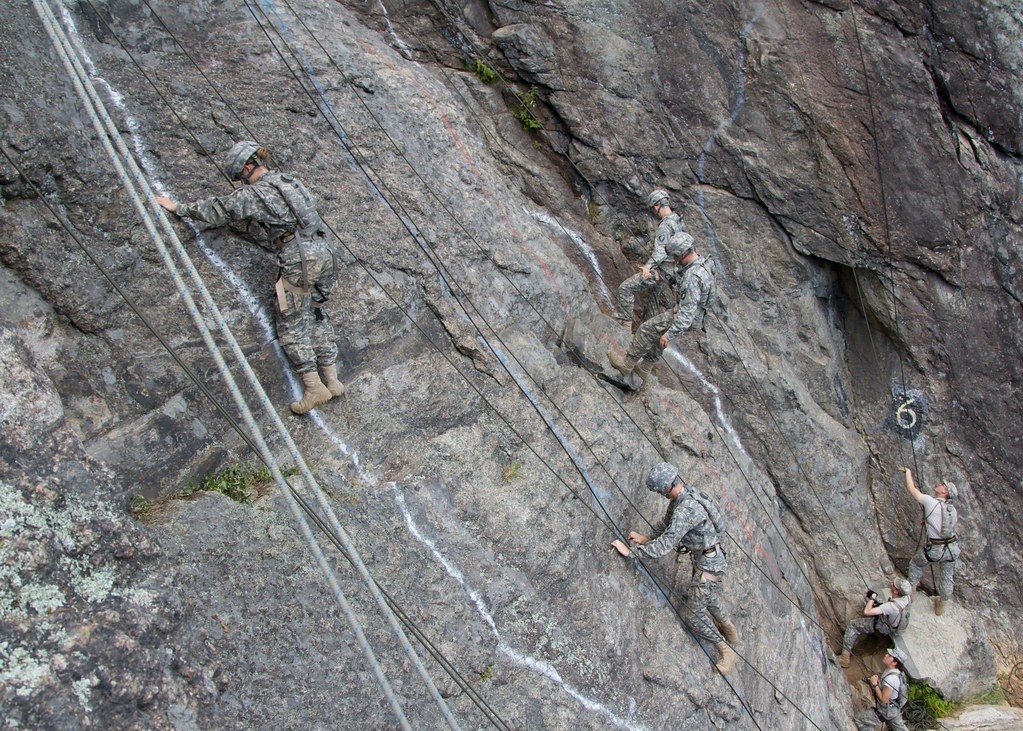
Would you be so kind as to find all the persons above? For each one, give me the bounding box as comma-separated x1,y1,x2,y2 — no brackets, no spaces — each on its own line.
897,460,962,617
613,187,687,346
605,230,719,394
153,141,345,413
834,576,913,671
852,647,912,731
609,460,741,676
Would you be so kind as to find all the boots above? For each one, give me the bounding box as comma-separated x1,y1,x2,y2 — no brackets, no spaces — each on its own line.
321,363,345,400
835,649,850,668
287,371,332,415
632,357,654,381
934,595,947,616
716,618,738,644
710,639,738,676
607,349,636,378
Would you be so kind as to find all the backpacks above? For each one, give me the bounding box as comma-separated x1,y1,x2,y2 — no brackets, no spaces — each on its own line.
887,595,912,636
882,671,910,708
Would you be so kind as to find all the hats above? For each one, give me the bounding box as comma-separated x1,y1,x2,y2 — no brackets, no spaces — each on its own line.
893,577,913,596
887,647,907,665
943,479,958,500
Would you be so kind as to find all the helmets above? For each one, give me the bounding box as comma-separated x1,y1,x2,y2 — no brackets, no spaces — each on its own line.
643,462,678,493
224,140,260,183
645,190,670,208
665,232,695,257
